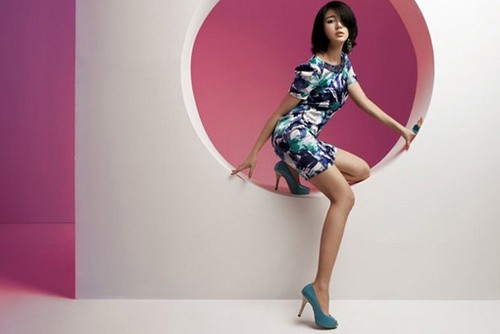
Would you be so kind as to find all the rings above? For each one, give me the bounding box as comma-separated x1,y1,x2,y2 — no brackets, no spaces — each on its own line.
412,124,420,134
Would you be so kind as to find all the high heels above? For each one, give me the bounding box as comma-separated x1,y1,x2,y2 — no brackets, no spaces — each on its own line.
274,160,311,194
298,284,338,328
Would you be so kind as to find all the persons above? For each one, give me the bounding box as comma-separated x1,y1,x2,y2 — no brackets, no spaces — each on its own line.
231,0,423,329
